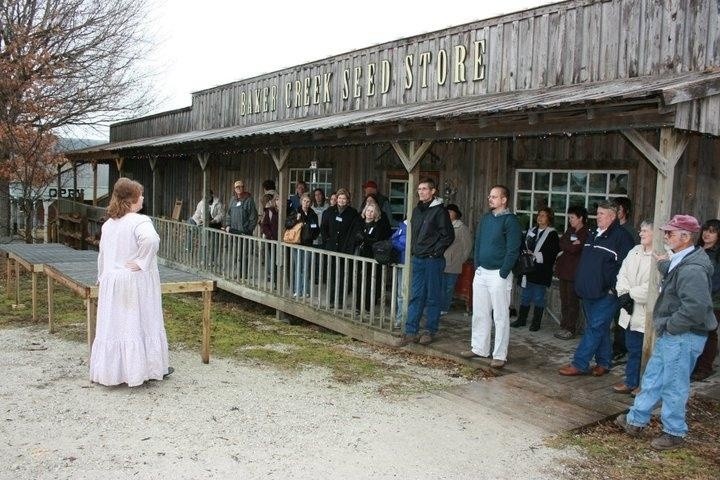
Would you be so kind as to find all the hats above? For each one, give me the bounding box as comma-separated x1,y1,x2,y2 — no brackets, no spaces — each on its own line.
234,181,243,189
659,214,700,232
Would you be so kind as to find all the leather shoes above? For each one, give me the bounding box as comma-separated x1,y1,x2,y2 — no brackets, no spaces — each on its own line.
613,384,640,398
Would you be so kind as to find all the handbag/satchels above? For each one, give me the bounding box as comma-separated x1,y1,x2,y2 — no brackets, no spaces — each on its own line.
283,223,304,244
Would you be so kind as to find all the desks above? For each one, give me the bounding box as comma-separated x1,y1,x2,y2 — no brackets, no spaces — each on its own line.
0,243,216,368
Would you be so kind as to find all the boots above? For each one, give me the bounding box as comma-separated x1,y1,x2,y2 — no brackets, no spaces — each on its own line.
510,305,543,331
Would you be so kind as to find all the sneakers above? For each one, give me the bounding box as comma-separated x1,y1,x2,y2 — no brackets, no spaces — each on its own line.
393,334,432,347
461,350,476,357
617,414,644,436
491,360,505,369
559,364,605,376
555,329,575,340
650,433,682,449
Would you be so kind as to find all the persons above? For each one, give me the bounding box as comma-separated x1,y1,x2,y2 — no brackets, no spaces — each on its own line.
185,179,390,315
88,177,174,388
508,206,585,339
618,215,718,451
392,183,472,347
459,182,522,368
552,197,719,393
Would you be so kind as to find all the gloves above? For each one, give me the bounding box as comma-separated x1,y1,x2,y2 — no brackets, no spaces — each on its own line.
618,293,634,315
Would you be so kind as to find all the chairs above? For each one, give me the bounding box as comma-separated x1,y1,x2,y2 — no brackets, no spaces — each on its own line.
157,199,182,221
